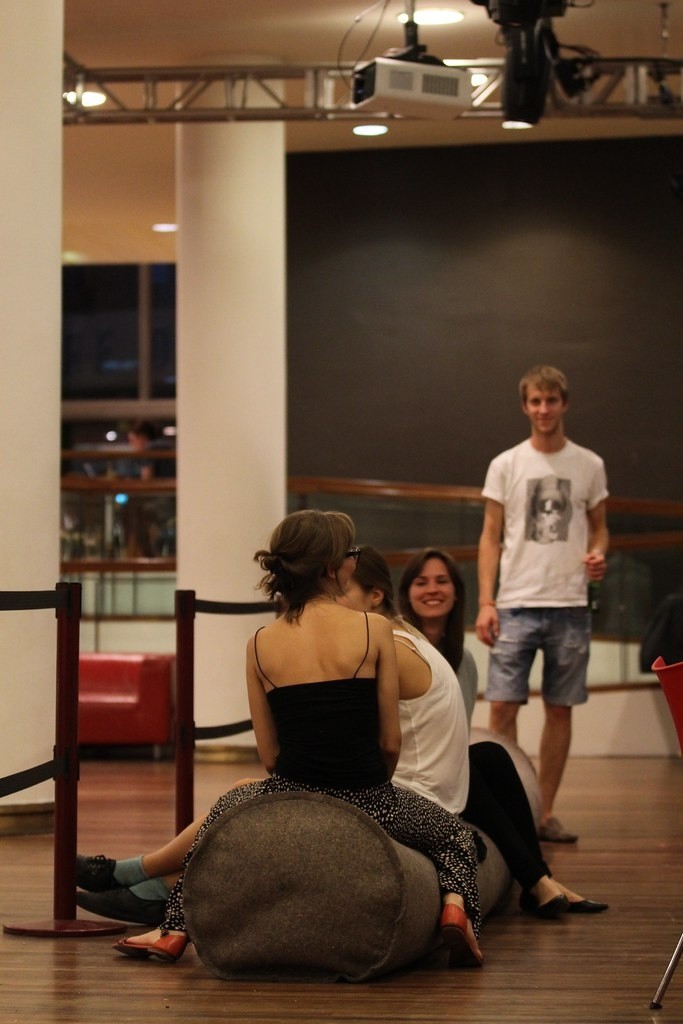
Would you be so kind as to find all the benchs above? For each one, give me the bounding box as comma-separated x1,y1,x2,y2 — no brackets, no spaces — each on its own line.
79,653,171,759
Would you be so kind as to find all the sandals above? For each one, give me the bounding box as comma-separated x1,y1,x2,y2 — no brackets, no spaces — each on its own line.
441,903,483,966
112,929,190,963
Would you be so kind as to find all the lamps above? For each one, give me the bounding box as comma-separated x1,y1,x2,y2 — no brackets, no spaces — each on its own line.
500,48,547,130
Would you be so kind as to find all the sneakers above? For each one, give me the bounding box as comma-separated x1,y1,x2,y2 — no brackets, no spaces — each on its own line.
538,817,578,842
76,886,167,925
77,854,126,892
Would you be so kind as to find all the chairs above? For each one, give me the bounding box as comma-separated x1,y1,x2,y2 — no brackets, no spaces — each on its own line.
651,656,683,1009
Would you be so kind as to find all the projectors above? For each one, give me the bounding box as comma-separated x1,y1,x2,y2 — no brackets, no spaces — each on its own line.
349,58,472,121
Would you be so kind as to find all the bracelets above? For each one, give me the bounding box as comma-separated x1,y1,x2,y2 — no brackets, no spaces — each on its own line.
480,601,495,609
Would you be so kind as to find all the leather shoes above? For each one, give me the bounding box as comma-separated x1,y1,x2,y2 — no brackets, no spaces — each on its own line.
519,888,609,912
536,895,569,916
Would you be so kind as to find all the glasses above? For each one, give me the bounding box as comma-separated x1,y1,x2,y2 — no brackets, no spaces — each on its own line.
343,546,361,565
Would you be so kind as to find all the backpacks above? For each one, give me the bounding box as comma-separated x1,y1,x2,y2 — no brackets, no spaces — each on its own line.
640,593,683,672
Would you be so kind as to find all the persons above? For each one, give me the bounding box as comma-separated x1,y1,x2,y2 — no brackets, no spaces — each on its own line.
398,547,611,916
73,545,471,927
111,511,488,967
475,365,610,843
